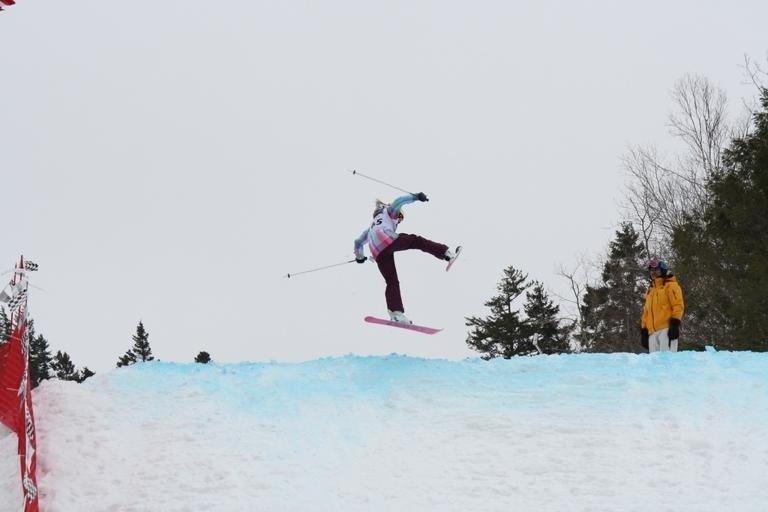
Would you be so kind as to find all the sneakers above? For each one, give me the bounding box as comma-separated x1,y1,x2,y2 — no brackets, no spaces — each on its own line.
388,312,414,325
444,240,465,264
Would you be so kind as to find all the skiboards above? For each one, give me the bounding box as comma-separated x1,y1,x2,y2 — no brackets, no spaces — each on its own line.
446,246,462,271
365,317,443,334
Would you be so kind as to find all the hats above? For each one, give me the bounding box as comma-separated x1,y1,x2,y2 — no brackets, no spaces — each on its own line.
399,207,405,221
647,259,669,280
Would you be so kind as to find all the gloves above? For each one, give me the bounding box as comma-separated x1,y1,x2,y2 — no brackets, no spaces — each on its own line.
638,327,652,350
416,191,428,203
667,316,681,341
355,256,368,265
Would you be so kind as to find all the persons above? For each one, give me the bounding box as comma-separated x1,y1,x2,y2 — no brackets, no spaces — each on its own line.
641,260,684,354
353,193,461,325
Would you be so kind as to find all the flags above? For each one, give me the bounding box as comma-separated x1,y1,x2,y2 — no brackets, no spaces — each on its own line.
0,304,38,512
